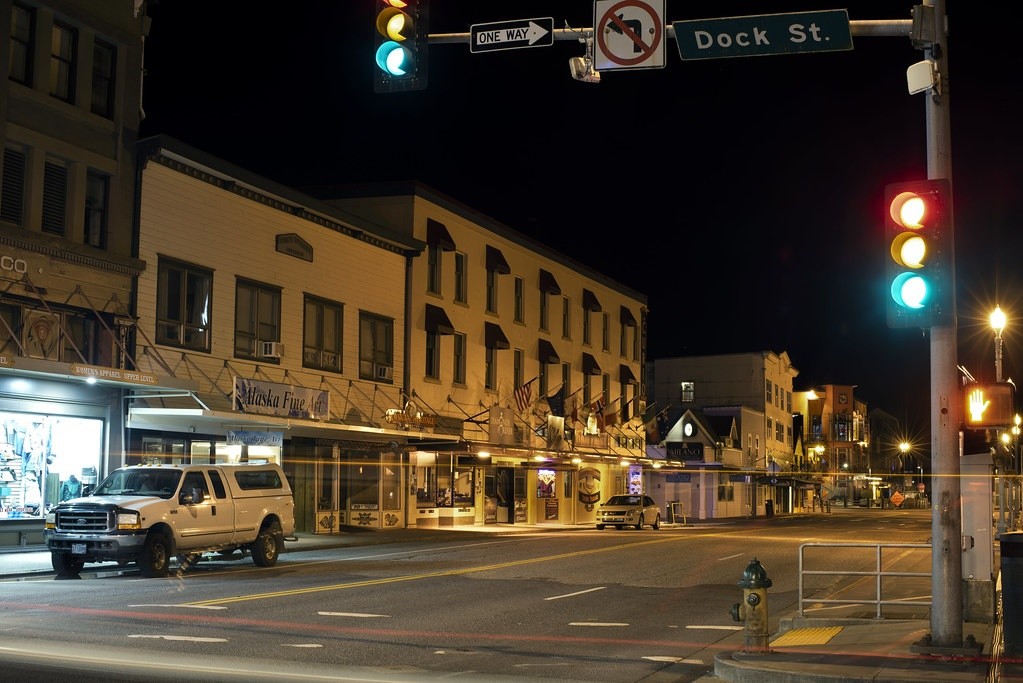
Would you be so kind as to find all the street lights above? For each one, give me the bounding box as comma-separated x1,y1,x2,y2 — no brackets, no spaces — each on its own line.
986,302,1012,535
1011,413,1022,503
899,442,910,509
812,444,823,472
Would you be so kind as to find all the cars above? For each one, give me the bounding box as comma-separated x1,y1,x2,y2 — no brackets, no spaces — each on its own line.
596,494,661,530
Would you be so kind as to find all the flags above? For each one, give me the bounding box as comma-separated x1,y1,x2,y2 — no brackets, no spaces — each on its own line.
514,382,669,436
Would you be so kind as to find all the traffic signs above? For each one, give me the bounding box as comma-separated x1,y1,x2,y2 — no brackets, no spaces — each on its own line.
470,17,554,54
672,8,854,62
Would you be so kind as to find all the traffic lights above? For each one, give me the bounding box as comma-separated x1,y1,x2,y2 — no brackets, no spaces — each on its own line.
373,0,428,92
961,381,1014,429
881,178,958,327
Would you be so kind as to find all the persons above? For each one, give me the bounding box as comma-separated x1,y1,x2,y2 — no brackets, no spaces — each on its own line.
23,419,49,477
60,472,83,502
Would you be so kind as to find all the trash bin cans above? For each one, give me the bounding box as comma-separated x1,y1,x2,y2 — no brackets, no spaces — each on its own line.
765,500,773,518
665,500,680,522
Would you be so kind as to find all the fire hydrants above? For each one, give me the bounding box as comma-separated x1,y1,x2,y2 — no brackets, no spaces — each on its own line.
729,557,773,653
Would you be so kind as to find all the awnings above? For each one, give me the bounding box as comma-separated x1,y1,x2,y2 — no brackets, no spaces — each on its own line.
582,352,602,376
484,322,510,350
620,363,637,385
620,305,637,327
583,288,603,312
426,218,457,253
538,338,560,365
424,302,455,336
538,267,562,296
485,245,512,275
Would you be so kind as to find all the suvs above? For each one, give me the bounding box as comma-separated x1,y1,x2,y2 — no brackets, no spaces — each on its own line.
44,462,298,579
